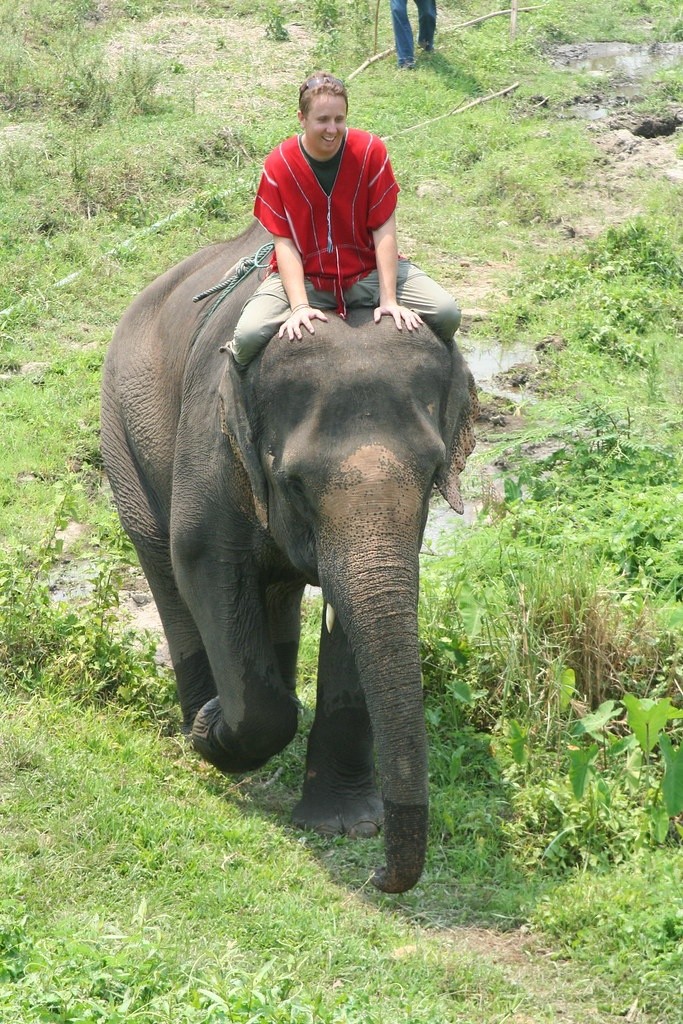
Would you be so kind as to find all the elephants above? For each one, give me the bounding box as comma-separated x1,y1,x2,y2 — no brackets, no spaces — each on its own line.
102,215,479,895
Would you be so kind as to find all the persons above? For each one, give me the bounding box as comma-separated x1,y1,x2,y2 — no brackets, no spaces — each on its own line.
390,0,436,69
228,73,462,364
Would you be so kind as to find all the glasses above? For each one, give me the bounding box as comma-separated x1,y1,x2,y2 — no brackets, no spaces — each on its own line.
299,77,344,104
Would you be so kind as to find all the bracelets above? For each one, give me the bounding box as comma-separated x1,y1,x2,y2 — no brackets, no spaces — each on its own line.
292,304,310,315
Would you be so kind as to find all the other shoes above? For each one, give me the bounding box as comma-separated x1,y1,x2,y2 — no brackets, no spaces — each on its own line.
401,61,418,72
420,46,434,60
